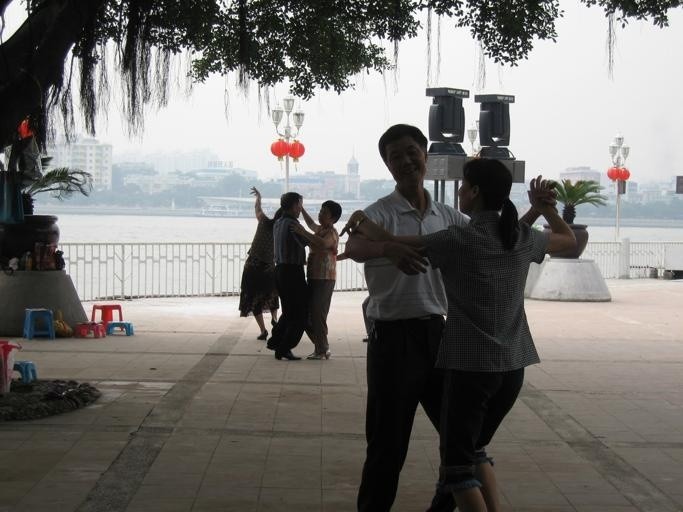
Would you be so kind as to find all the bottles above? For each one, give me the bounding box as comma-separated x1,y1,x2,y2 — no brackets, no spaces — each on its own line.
55,309,63,321
9,244,65,272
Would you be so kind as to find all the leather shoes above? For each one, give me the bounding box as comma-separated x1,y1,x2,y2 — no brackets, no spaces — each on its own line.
257,331,268,340
267,344,302,360
307,351,331,360
271,317,277,327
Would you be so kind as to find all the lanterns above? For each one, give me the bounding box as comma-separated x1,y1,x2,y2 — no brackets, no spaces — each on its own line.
619,167,630,180
606,167,619,193
19,120,32,138
289,139,305,172
271,138,289,171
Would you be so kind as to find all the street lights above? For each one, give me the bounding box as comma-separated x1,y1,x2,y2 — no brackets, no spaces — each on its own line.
606,135,629,244
271,94,304,195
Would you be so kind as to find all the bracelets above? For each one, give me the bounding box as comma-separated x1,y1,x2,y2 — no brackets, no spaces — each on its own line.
356,213,366,230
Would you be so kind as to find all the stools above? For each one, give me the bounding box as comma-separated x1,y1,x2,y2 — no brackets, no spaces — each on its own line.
0,340,22,392
91,304,124,331
106,321,133,336
14,361,37,383
23,309,55,340
75,325,105,337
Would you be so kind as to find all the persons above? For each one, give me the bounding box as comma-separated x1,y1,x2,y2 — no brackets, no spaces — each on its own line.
343,124,559,512
338,154,577,512
289,200,342,361
237,185,282,341
265,192,308,361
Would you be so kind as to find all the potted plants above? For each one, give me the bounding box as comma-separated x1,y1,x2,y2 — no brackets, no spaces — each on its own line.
542,178,610,258
0,153,96,265
